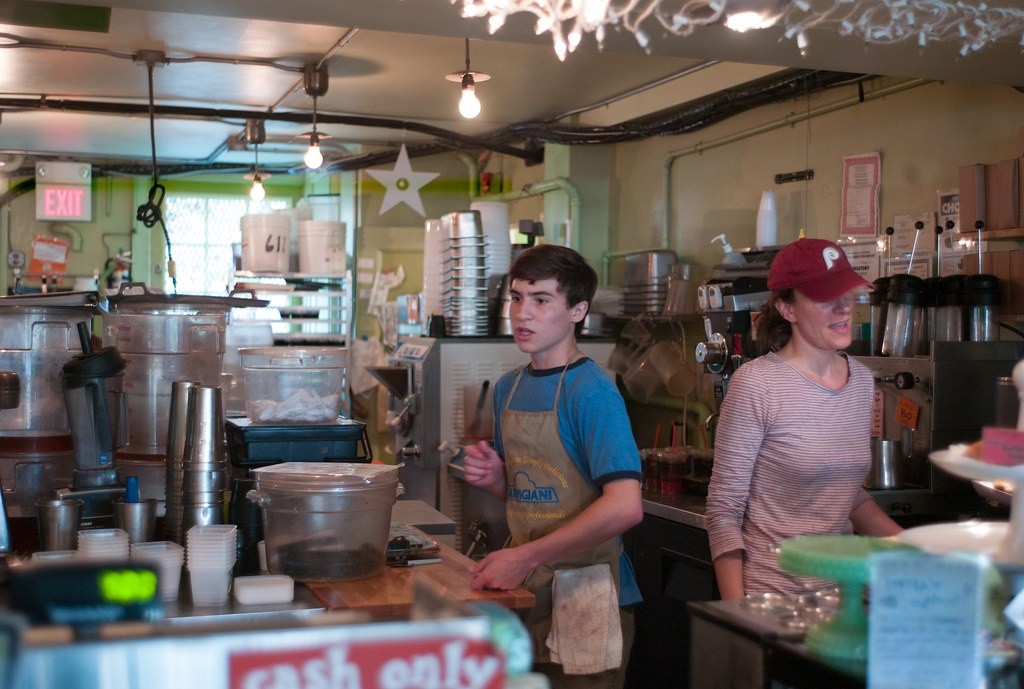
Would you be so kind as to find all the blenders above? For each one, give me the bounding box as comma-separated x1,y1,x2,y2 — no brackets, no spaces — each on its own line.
56,322,140,525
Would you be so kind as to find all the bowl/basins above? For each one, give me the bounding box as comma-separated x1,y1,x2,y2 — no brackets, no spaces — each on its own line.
33,525,294,606
580,248,678,338
419,201,516,336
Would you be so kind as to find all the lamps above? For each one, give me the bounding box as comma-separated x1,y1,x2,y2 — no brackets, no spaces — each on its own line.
444,39,492,122
243,144,274,203
293,97,332,171
33,161,94,226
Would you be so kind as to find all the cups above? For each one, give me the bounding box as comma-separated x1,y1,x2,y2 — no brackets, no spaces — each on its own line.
34,501,83,551
609,318,715,507
163,382,228,547
862,438,905,489
111,497,159,543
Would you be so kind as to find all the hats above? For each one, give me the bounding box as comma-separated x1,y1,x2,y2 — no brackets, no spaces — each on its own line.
768,238,875,304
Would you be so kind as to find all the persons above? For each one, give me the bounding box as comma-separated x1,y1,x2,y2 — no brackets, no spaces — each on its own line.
705,239,905,603
460,244,644,689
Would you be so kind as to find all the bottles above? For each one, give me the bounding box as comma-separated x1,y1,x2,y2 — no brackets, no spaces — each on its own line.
756,187,779,251
661,265,699,317
870,272,1001,357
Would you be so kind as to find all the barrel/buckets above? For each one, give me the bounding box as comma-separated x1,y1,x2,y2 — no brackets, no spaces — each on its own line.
1,306,93,518
239,214,290,273
297,219,347,277
246,461,404,584
227,320,350,425
93,309,226,517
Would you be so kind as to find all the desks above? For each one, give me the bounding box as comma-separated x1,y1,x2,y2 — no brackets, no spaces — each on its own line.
687,594,866,689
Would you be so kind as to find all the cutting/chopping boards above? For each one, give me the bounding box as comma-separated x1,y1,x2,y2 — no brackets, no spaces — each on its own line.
305,522,537,620
